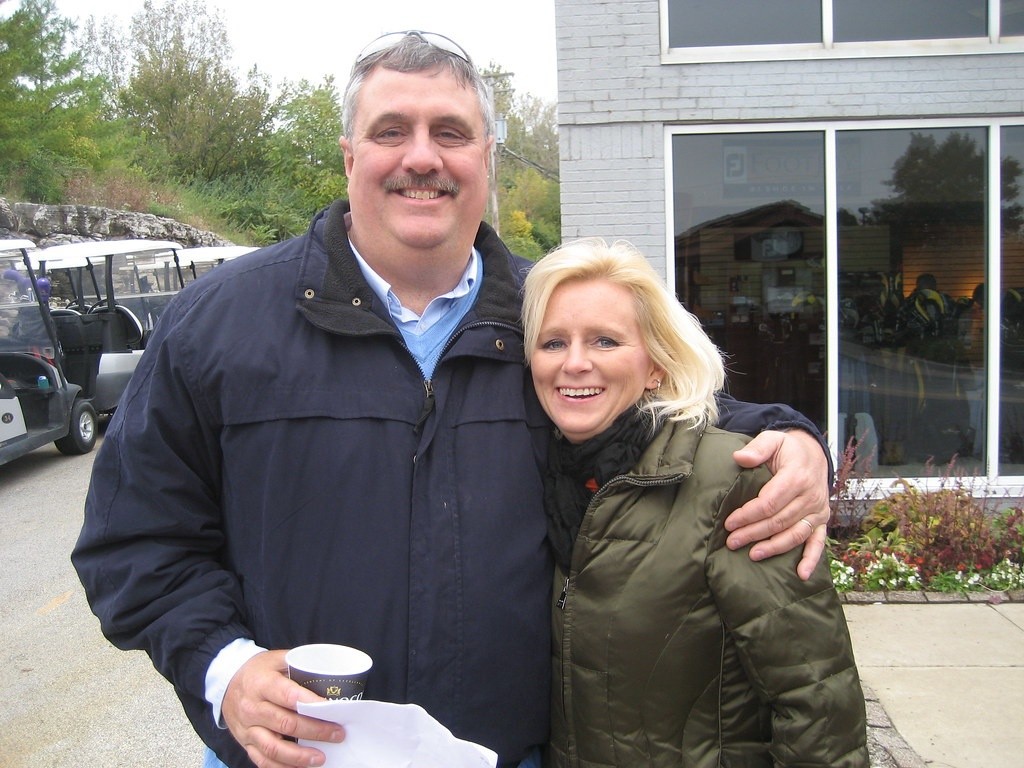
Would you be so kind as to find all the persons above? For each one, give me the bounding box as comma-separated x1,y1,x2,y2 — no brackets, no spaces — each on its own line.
895,274,955,427
521,235,871,768
69,29,835,768
957,283,984,461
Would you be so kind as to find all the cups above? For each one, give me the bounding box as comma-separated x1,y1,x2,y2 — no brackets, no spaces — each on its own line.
284,644,373,700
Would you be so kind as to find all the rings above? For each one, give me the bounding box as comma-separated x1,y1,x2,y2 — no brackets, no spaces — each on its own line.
802,518,814,536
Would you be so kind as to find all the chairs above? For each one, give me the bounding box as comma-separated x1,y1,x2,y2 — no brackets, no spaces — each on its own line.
50,298,143,351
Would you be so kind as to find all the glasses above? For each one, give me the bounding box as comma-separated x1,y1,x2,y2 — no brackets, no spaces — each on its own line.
354,31,473,68
